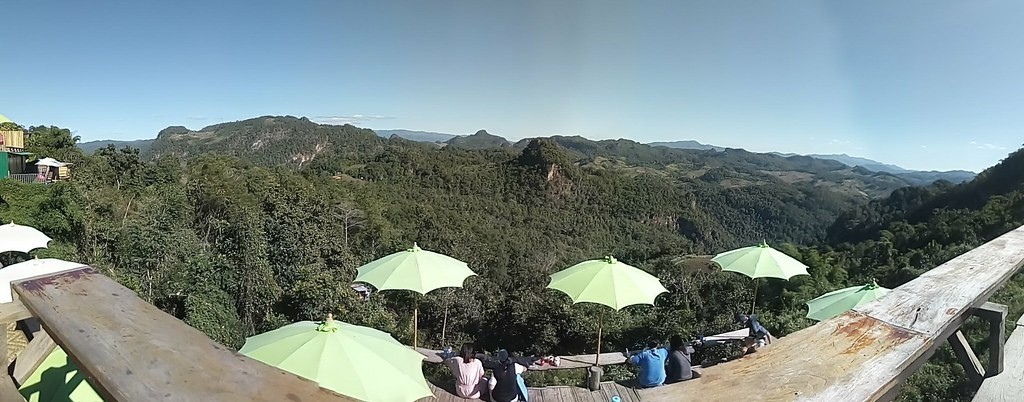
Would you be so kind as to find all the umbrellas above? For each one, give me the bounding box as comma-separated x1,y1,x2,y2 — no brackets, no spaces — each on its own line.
545,253,670,367
804,278,893,322
352,241,478,346
1,219,53,261
0,256,90,283
710,238,810,315
237,312,437,402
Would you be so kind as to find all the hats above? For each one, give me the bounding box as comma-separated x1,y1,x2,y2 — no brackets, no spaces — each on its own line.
670,335,682,346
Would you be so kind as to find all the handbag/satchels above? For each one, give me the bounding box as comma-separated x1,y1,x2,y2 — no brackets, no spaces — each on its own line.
691,369,702,379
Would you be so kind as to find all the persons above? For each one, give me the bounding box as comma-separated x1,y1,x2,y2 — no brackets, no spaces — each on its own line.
626,337,669,387
666,333,695,380
739,314,772,345
488,349,528,402
446,342,484,399
